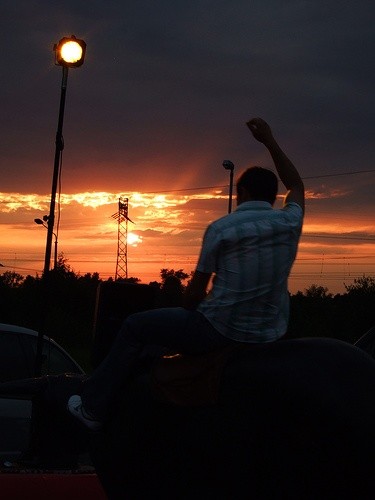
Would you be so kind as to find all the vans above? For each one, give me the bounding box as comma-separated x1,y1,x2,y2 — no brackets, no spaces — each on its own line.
0,322,89,466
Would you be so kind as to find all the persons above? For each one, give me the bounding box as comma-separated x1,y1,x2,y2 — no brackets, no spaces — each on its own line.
67,117,305,428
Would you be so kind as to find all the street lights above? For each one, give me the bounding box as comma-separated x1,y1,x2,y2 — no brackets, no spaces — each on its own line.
221,159,236,211
33,34,86,377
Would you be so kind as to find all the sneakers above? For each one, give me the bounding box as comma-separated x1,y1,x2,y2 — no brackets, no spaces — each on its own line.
68,395,104,432
